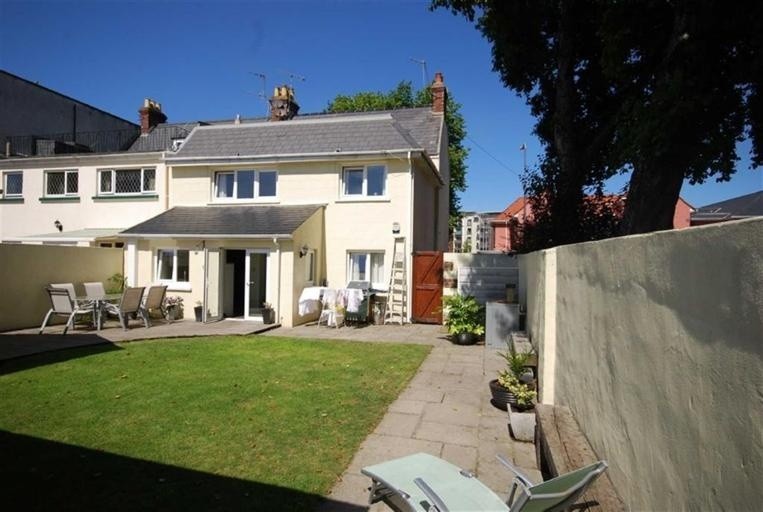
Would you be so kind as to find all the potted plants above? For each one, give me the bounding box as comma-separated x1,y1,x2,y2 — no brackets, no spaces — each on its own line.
436,295,485,344
262,303,274,324
489,339,539,410
194,301,202,322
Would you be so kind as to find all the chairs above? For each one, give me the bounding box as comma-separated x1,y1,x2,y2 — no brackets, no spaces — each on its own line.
360,453,608,512
36,278,168,337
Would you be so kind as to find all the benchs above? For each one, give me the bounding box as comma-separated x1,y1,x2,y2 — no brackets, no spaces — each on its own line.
535,402,622,511
509,330,537,368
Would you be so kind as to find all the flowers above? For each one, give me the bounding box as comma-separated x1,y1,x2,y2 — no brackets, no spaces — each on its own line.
164,295,177,309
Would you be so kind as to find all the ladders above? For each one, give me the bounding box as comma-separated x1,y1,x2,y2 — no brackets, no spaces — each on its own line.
384,237,406,326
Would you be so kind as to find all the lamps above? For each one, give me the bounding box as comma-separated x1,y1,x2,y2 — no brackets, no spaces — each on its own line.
54,220,62,231
299,244,309,258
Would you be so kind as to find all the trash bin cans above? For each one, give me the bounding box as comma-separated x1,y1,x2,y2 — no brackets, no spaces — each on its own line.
371,302,386,325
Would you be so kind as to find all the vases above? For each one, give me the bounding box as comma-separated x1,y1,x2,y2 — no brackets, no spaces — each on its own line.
166,309,176,321
